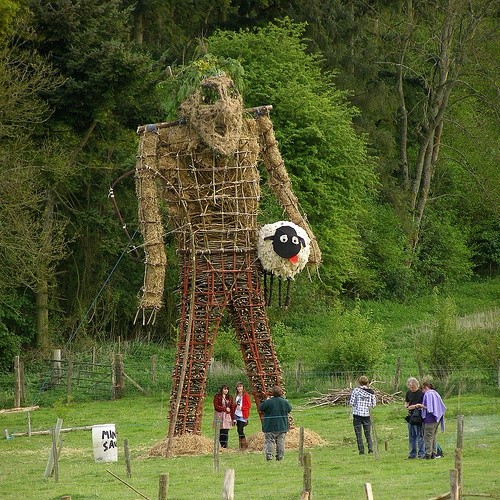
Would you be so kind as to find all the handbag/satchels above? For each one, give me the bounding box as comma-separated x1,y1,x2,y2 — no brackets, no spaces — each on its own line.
244,420,248,426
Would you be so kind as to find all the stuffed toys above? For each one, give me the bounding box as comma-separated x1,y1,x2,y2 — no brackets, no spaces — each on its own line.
257,220,311,282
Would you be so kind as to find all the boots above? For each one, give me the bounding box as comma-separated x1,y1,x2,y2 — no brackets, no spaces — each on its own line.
239,438,248,450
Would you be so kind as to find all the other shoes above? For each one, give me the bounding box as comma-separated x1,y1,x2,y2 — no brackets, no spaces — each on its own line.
418,456,430,459
408,456,415,458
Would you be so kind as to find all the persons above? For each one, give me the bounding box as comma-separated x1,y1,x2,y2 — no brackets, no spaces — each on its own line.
349,375,377,455
233,381,251,450
213,384,237,448
415,382,447,459
405,377,426,459
260,386,292,461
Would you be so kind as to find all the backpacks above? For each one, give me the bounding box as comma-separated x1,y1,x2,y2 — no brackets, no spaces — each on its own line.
409,409,424,438
437,443,442,456
